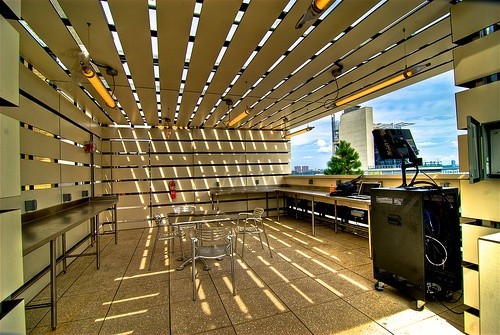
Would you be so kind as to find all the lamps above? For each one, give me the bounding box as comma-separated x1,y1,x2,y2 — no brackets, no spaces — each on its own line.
282,109,314,140
223,98,252,128
80,22,117,110
294,0,337,29
324,29,432,109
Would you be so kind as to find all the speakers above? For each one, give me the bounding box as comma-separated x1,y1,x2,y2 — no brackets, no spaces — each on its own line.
370,187,463,310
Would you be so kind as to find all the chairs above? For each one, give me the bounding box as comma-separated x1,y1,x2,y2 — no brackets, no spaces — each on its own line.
148,206,273,303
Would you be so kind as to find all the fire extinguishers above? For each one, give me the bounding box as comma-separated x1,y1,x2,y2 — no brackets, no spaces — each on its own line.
169,181,176,199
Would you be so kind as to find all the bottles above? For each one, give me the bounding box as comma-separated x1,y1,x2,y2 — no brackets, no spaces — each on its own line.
330,184,335,193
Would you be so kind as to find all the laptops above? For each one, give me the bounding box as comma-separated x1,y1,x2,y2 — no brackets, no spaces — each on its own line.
349,181,381,201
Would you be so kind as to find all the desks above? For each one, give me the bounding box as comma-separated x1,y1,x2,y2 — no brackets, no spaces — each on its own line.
167,184,371,272
22,196,119,331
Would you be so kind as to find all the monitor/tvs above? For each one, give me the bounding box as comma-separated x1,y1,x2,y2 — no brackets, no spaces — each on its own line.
372,129,418,188
